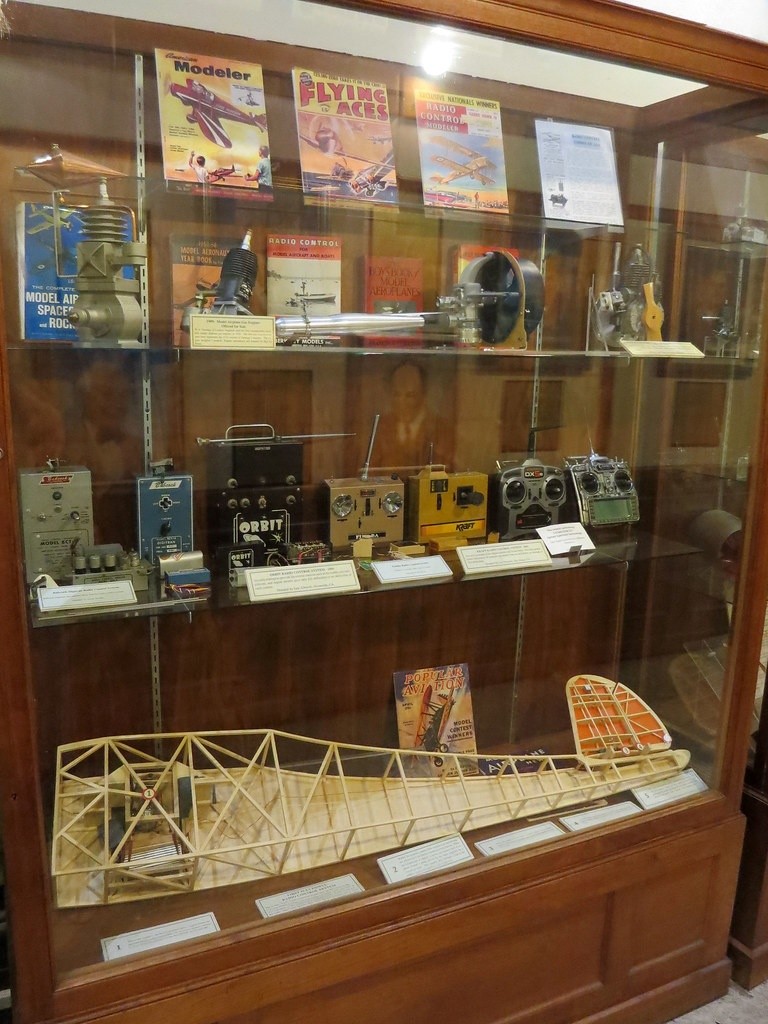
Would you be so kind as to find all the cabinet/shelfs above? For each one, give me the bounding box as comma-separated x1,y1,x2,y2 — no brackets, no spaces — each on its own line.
2,0,766,1024
